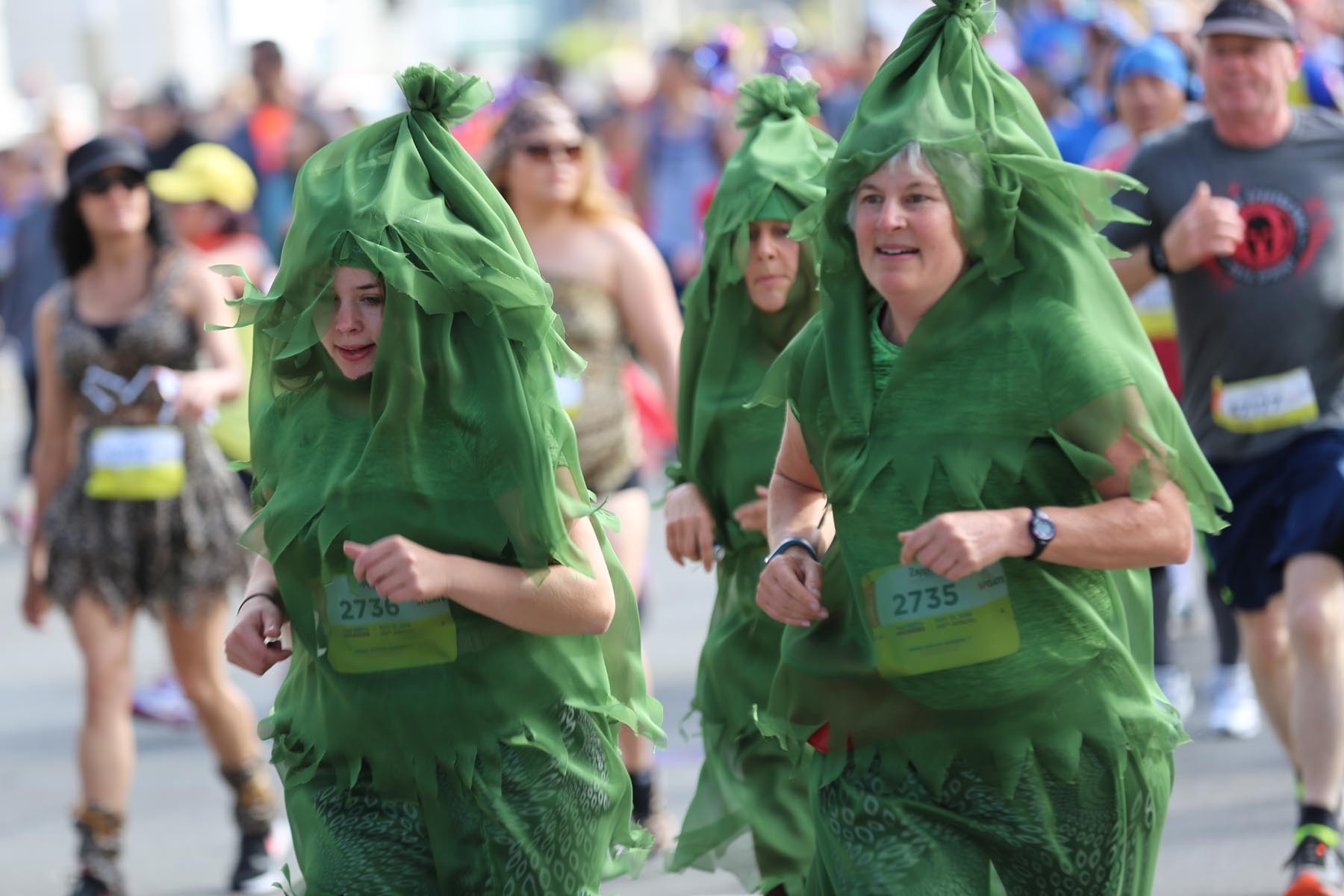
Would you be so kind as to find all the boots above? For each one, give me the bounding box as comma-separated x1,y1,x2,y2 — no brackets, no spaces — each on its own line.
70,806,129,896
223,771,304,894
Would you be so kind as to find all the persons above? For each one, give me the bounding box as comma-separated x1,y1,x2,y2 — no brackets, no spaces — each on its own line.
472,82,686,860
1094,0,1344,896
224,64,616,896
0,0,1344,744
664,0,1191,896
22,132,292,896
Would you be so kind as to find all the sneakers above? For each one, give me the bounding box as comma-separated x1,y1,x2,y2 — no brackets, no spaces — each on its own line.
1282,807,1344,896
1293,769,1305,804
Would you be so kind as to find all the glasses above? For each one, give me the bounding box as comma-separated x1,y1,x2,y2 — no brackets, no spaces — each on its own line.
525,146,581,163
80,171,141,193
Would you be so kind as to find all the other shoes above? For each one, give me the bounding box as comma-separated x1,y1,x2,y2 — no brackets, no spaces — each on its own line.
640,815,671,855
1154,666,1195,722
1203,663,1262,738
134,672,201,723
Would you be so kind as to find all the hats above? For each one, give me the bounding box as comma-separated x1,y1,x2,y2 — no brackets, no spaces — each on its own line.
66,137,148,182
1196,3,1296,44
145,142,257,215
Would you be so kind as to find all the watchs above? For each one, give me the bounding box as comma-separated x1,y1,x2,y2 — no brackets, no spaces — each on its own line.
1024,505,1057,561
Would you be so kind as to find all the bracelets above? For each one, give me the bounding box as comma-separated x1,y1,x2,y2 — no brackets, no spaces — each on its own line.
1148,237,1170,274
764,537,819,566
236,593,284,617
